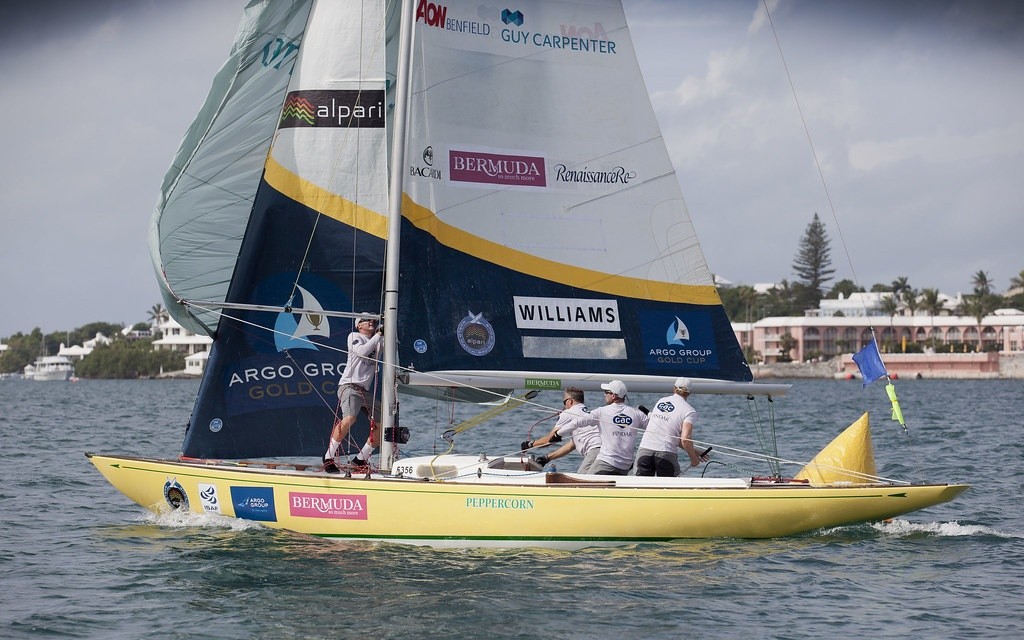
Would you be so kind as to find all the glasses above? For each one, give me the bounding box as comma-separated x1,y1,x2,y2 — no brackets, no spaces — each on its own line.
563,398,571,405
358,319,375,323
604,390,612,394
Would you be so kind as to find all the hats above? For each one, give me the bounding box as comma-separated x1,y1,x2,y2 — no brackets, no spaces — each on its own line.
355,312,376,329
600,379,627,399
674,377,692,394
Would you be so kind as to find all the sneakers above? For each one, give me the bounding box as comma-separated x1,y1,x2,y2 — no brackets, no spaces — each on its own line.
322,455,340,474
348,457,369,469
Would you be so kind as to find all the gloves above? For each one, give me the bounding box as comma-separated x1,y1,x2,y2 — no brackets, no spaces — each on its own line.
521,440,535,453
377,325,384,336
548,430,562,443
536,453,550,467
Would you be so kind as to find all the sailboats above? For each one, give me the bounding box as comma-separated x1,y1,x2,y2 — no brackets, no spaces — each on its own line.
85,0,970,544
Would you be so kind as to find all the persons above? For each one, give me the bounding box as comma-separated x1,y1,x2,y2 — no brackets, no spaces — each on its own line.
322,312,385,474
521,386,603,475
548,379,650,476
633,377,711,477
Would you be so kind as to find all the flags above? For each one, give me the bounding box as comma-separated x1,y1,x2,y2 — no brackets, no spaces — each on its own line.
851,337,887,389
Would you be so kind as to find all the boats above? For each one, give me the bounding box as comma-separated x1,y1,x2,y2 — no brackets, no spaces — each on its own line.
31,356,74,382
